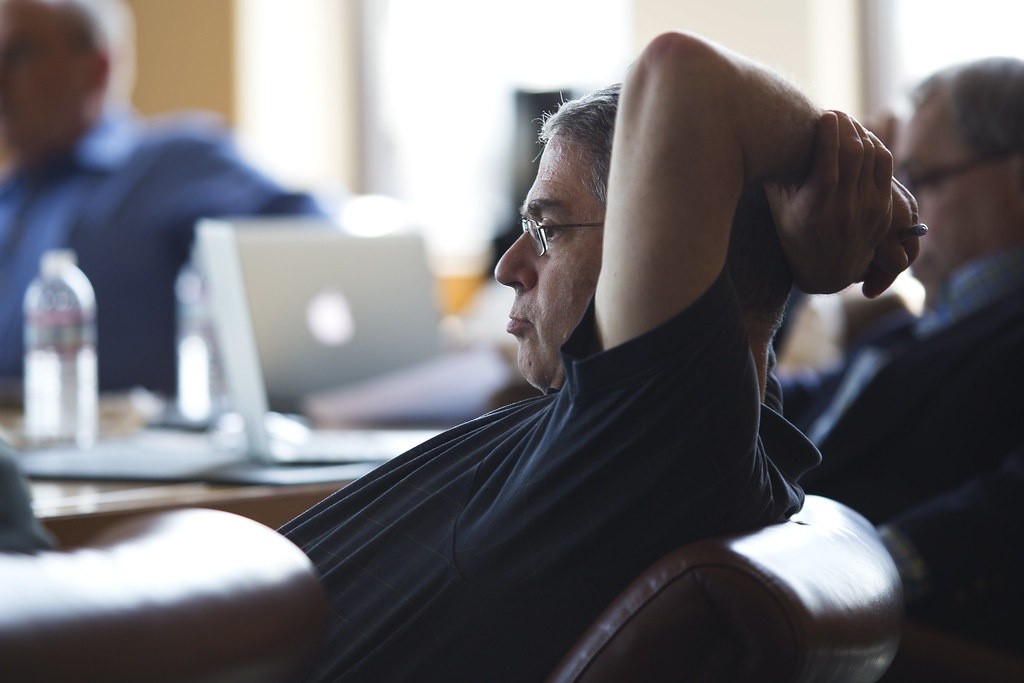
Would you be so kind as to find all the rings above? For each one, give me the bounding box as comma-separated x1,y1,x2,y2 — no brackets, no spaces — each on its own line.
898,223,928,241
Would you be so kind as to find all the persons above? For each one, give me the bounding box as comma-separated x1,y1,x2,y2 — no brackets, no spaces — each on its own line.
277,33,1024,683
0,0,324,683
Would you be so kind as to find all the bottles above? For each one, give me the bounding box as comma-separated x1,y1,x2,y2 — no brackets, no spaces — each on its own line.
19,251,98,448
171,247,228,425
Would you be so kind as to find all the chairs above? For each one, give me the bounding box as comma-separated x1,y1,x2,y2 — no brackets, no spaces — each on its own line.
0,505,320,683
552,491,908,683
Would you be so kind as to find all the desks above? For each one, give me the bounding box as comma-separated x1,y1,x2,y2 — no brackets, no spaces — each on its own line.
0,393,392,546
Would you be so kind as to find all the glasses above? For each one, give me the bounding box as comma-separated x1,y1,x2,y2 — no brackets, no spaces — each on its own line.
522,217,604,256
893,149,1024,200
